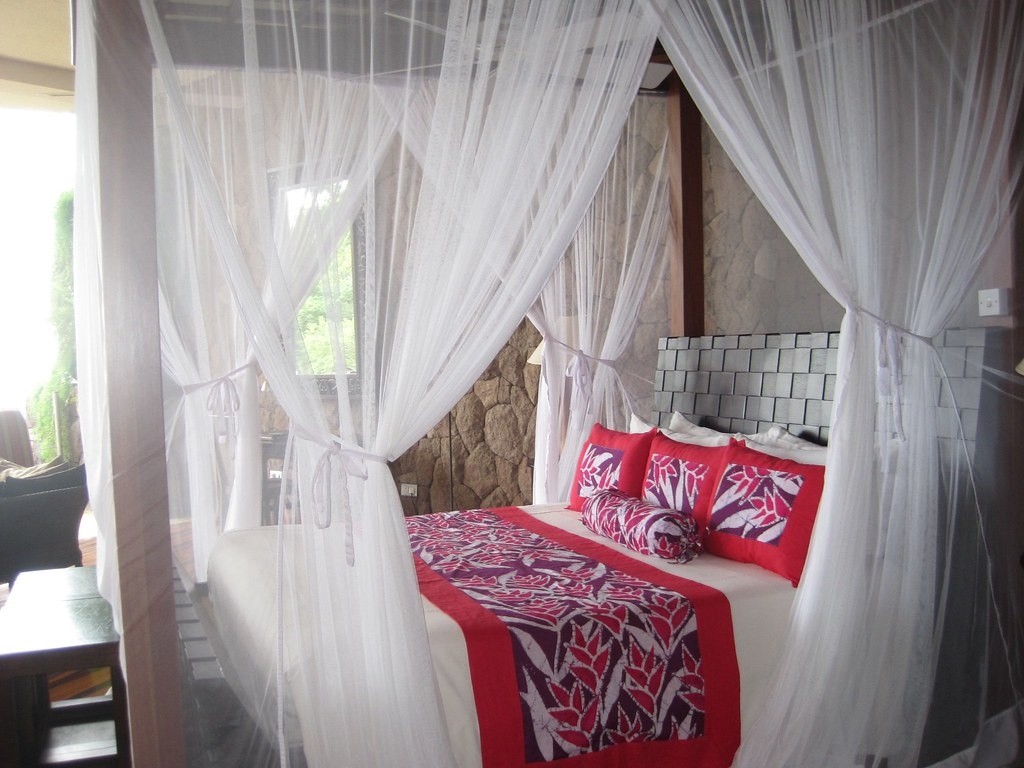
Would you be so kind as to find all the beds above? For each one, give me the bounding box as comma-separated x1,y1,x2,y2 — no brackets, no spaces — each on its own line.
101,490,900,768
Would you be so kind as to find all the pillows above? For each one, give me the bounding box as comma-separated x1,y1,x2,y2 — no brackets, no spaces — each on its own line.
561,425,654,513
705,438,827,589
733,433,829,469
627,414,730,448
763,427,828,451
668,410,735,438
641,429,728,556
579,488,703,566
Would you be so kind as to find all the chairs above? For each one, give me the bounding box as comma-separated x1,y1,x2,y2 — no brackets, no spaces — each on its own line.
0,486,91,592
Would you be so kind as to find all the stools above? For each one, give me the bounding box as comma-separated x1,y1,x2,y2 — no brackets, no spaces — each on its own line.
2,566,132,768
